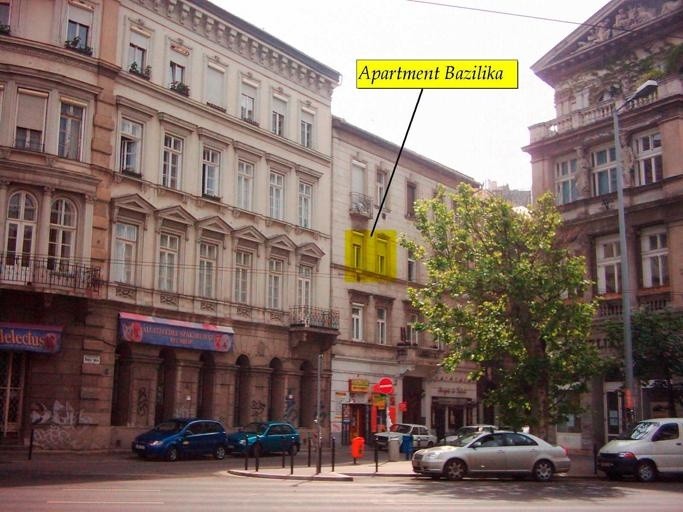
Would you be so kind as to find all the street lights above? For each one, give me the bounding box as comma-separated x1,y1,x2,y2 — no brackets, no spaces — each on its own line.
612,79,659,414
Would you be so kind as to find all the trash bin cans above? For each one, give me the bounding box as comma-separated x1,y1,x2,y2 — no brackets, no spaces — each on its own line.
351,436,365,459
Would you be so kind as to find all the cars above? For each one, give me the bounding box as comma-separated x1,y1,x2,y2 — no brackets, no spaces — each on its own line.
129,417,301,461
373,422,570,482
597,416,682,482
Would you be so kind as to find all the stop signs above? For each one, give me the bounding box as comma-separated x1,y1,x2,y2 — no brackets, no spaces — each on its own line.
379,378,392,394
